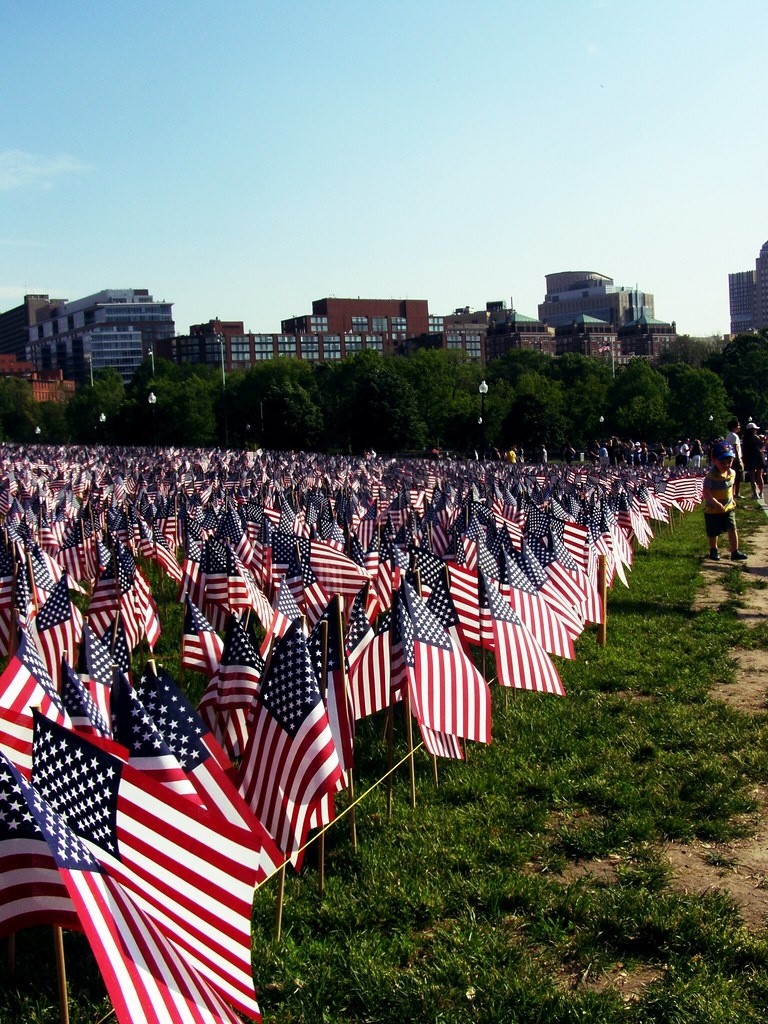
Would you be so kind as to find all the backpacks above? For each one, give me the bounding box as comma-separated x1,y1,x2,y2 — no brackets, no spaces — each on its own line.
564,448,574,460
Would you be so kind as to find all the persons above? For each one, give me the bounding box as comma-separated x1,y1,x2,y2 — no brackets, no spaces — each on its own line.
364,434,726,466
704,442,747,560
758,431,768,485
726,418,746,499
742,422,768,499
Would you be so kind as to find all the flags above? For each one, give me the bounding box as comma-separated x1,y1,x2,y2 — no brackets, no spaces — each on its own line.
0,468,704,1024
0,441,541,467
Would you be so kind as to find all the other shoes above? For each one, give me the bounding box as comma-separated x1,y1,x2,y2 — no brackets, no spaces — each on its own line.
737,496,746,499
751,493,760,499
731,550,747,561
710,548,720,560
759,493,764,499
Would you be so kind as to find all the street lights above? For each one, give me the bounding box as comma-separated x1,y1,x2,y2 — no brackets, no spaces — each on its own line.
99,413,106,445
478,417,482,431
216,335,229,448
147,349,155,377
599,416,604,444
87,356,94,386
148,392,157,447
478,380,488,461
708,415,713,441
35,427,41,444
748,416,753,423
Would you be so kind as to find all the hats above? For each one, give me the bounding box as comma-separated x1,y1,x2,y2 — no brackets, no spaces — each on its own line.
747,423,760,429
635,442,640,446
713,442,736,459
